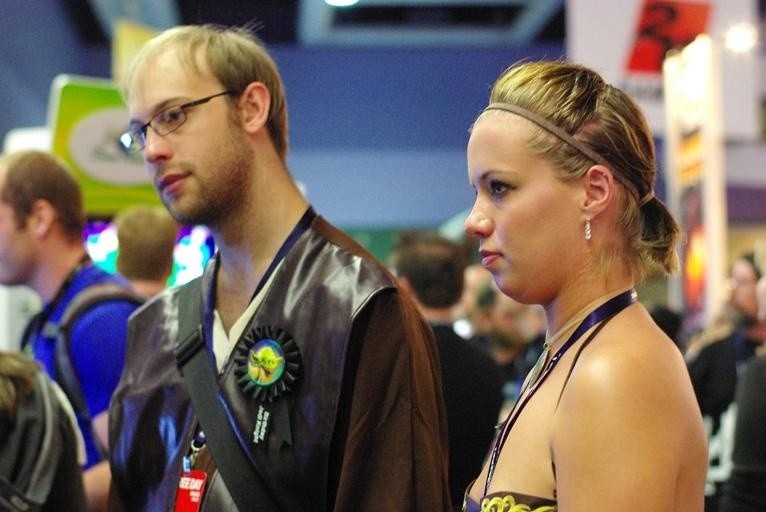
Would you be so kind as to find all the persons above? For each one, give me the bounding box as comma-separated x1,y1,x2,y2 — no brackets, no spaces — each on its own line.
645,252,766,512
0,151,177,510
462,62,709,512
106,26,454,512
389,227,548,511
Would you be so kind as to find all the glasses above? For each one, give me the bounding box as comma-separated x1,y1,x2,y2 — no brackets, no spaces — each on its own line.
117,89,242,159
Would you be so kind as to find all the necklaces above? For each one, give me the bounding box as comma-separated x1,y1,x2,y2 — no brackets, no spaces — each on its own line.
528,284,634,389
